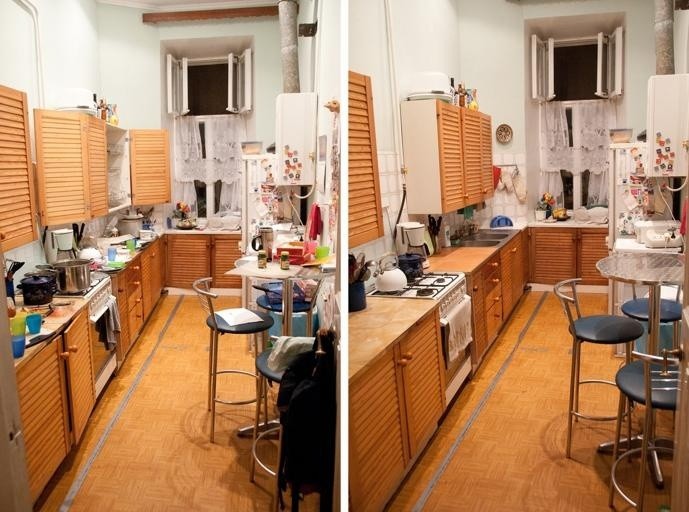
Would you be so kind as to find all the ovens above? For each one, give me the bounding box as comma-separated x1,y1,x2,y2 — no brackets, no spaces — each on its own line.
440,295,474,407
90,295,119,401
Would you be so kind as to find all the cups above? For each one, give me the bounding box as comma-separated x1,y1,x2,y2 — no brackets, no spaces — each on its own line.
26,314,42,335
315,246,330,260
107,247,116,262
11,335,26,358
10,319,26,337
126,239,136,257
306,242,316,254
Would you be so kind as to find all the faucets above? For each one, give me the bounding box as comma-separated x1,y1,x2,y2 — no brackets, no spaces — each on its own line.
463,223,478,237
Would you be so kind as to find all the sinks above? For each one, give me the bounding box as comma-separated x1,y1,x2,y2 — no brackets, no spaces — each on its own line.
454,240,500,247
467,233,508,239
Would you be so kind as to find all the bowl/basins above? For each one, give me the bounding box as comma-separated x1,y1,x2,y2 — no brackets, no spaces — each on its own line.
50,300,73,317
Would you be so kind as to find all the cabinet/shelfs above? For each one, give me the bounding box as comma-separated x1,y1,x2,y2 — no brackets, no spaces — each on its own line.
348,295,447,512
424,247,504,366
528,227,609,286
159,233,167,292
128,254,144,344
33,107,172,227
350,71,385,248
108,268,131,363
141,240,165,323
165,233,242,288
498,230,524,321
400,100,494,215
0,85,38,254
6,295,97,508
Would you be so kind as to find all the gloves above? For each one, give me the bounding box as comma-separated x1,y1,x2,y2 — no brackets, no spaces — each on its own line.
513,172,527,202
498,169,514,193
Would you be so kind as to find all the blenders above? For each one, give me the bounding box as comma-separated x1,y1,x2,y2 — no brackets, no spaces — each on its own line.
48,228,77,263
396,221,432,269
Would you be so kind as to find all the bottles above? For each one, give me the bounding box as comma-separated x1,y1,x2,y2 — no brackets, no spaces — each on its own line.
91,94,120,126
258,251,266,269
448,77,478,106
281,252,289,269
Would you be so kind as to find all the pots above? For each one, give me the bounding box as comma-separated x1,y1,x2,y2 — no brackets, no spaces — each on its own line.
53,258,93,293
16,274,58,307
119,214,144,238
395,252,426,281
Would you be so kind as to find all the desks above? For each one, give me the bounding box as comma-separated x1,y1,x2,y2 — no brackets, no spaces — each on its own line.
595,251,685,460
233,256,336,439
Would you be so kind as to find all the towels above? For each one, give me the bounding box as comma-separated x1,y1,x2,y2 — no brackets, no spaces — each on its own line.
103,295,122,351
308,202,322,241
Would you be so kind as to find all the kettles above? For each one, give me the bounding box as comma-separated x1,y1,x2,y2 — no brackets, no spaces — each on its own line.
252,227,273,262
376,255,409,295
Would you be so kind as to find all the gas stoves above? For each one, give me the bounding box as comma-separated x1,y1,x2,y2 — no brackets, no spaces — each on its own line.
371,269,459,302
11,300,54,320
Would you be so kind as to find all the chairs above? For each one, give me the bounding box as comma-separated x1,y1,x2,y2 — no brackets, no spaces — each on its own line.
249,335,316,511
608,351,680,512
252,279,324,398
621,284,682,422
193,277,268,443
553,278,645,463
